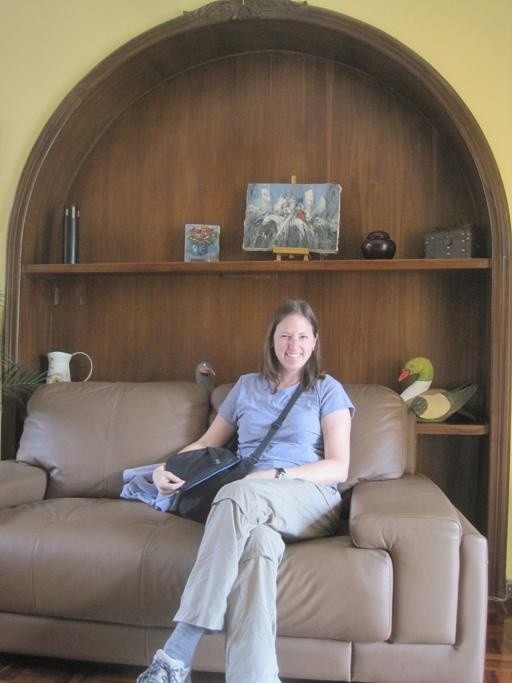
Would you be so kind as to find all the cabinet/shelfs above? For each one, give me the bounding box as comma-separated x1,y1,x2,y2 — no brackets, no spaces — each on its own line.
0,1,509,608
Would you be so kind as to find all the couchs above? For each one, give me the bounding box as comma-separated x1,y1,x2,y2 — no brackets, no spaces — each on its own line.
1,379,488,682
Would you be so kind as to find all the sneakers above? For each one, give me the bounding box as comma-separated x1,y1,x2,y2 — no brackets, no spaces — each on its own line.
136,649,192,683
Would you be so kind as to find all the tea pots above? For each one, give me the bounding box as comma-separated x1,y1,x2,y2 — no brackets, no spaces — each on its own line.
46,351,94,383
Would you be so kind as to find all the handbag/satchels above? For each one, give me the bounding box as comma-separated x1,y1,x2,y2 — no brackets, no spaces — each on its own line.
164,447,252,524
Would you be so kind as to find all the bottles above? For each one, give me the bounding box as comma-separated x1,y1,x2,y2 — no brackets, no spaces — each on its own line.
362,229,396,259
63,203,79,264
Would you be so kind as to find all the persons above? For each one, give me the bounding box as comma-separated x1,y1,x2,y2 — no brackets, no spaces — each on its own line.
135,299,355,683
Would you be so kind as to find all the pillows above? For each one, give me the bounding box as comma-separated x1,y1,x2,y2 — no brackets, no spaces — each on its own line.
13,378,209,499
208,378,409,494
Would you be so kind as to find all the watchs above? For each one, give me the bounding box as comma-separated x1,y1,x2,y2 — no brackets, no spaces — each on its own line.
276,468,287,479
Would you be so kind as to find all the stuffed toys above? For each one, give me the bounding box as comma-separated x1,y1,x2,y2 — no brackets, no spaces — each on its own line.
398,357,477,423
